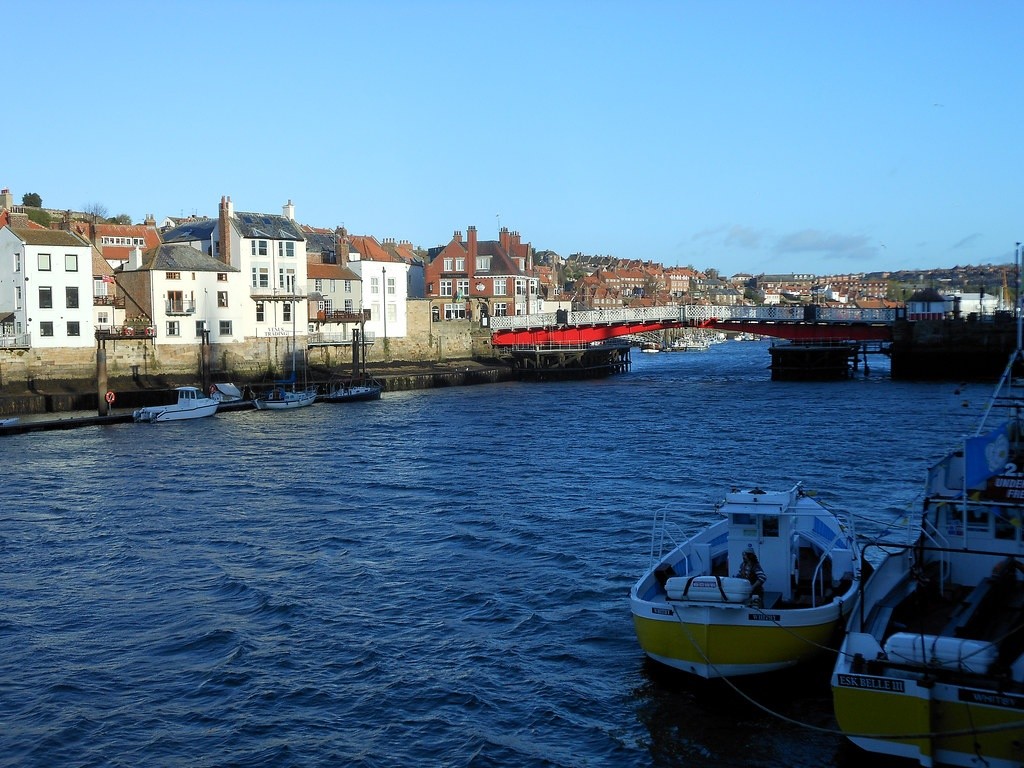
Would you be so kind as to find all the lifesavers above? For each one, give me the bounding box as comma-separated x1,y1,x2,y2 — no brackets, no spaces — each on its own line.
124,327,135,337
209,384,217,394
145,327,154,336
105,391,115,403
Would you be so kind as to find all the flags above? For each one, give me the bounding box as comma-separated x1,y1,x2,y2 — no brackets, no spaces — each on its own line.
966,421,1009,488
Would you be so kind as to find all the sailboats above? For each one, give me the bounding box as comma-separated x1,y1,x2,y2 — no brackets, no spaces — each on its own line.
250,278,387,410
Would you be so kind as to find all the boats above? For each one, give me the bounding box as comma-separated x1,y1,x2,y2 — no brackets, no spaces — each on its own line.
629,481,860,692
133,383,267,424
830,351,1023,768
642,349,659,353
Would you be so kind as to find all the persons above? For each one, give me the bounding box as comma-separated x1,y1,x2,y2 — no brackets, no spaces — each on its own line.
481,312,485,321
736,548,766,609
598,305,602,320
573,306,576,311
278,387,286,401
